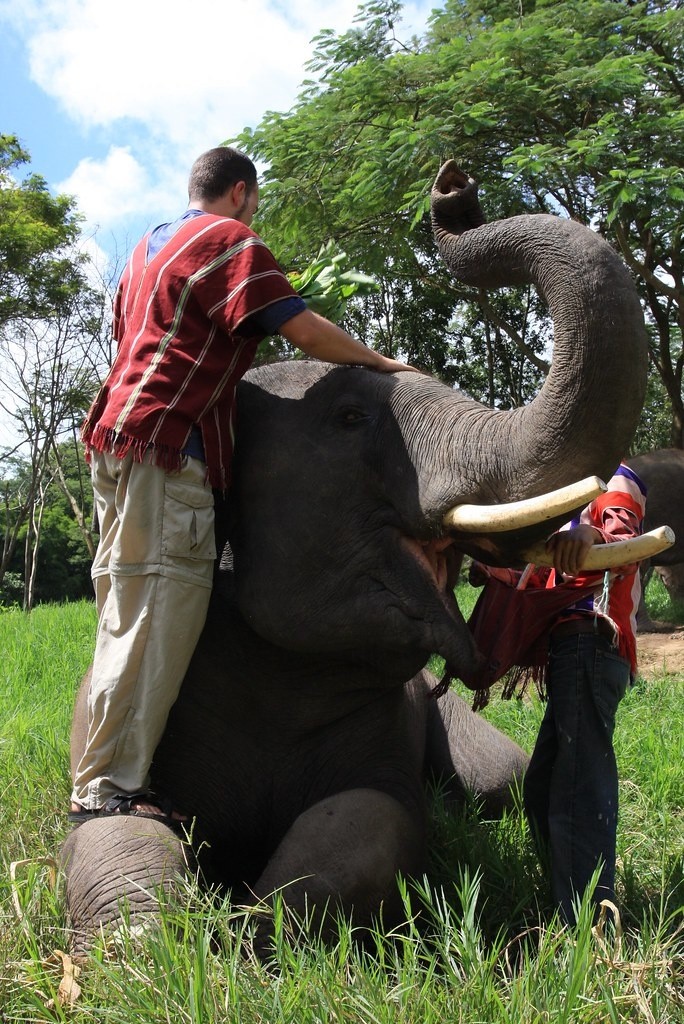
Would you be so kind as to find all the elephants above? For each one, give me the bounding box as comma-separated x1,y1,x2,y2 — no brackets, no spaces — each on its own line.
64,154,683,999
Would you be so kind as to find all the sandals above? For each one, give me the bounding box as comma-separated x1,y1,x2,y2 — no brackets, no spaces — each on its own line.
67,806,101,822
98,793,190,827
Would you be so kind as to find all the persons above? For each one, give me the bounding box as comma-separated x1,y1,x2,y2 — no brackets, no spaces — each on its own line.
469,455,647,941
67,146,420,823
